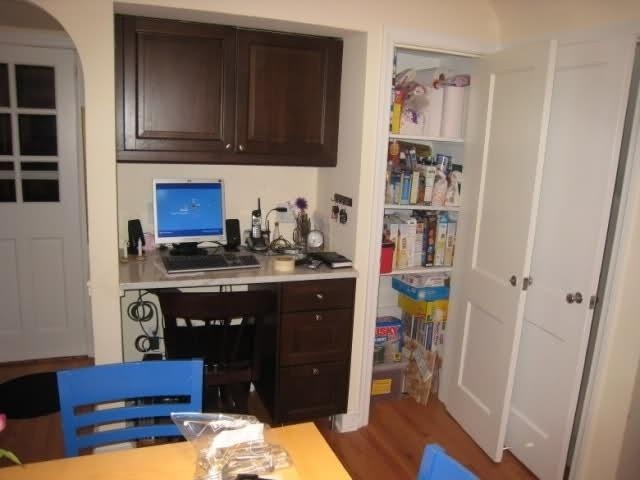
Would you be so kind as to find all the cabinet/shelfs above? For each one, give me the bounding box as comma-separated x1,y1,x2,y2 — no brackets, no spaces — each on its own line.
115,13,343,167
379,43,473,277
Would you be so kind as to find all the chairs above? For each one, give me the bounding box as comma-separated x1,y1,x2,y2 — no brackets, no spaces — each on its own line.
417,444,480,480
56,358,204,459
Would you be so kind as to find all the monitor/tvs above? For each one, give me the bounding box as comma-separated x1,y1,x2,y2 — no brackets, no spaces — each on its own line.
152,178,226,255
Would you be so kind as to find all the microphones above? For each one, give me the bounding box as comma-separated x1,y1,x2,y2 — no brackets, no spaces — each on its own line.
264,206,289,231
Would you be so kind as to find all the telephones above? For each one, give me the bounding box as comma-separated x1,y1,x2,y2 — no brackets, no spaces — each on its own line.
247,197,269,251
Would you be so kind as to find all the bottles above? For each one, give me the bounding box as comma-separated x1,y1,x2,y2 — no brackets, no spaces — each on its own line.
380,240,395,273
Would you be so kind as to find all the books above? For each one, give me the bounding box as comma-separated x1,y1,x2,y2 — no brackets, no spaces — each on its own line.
314,251,353,269
384,212,456,269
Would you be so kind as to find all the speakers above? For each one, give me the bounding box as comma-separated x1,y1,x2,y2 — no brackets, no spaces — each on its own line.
225,218,241,248
127,218,146,247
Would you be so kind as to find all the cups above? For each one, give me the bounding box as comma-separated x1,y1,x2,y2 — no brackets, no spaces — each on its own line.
142,233,156,252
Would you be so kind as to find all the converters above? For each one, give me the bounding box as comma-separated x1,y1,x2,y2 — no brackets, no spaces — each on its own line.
148,335,158,350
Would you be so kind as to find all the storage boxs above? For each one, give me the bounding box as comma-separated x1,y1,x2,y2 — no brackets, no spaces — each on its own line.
370,353,410,402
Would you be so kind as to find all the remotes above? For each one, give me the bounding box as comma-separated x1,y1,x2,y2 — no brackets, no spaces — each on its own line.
307,260,321,270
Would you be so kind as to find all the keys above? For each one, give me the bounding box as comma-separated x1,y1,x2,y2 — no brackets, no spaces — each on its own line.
330,205,348,224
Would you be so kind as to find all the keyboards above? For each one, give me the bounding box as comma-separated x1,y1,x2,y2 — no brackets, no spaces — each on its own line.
161,255,261,274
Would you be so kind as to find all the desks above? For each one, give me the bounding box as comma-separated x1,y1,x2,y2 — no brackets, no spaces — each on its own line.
0,421,352,480
117,244,359,428
